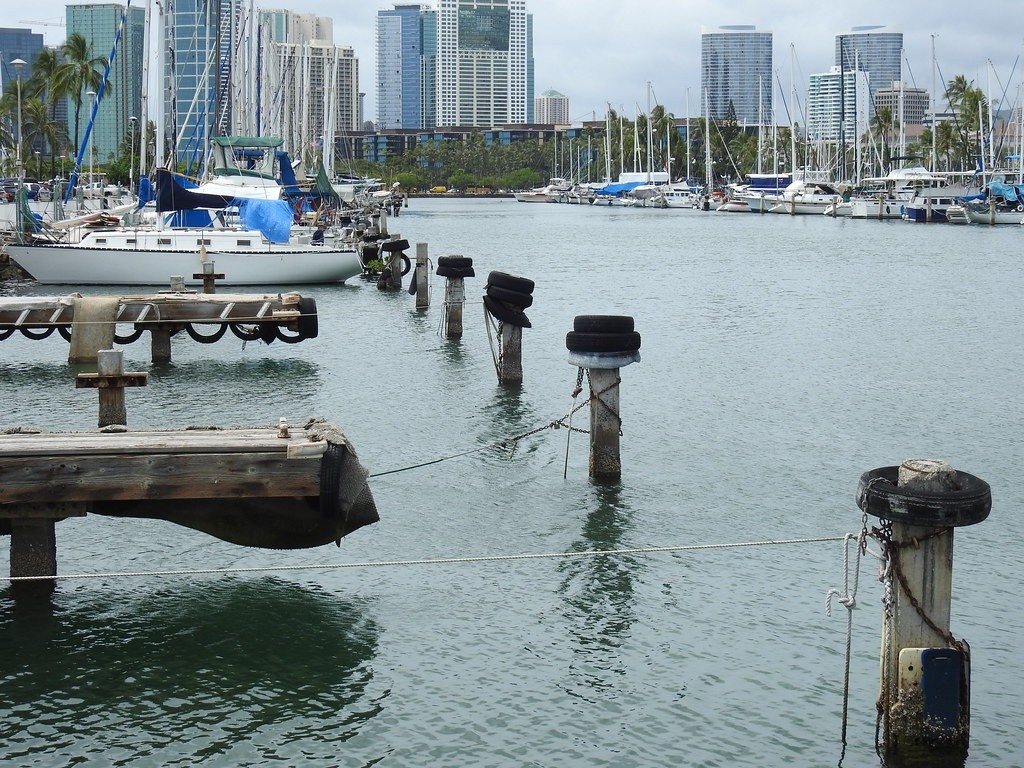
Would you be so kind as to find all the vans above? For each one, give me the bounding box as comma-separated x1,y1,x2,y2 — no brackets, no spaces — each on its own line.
429,186,447,193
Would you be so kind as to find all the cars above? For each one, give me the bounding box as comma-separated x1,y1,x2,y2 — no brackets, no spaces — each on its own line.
447,188,460,194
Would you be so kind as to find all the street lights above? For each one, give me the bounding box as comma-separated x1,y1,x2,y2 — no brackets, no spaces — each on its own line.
127,116,138,197
6,57,28,235
85,90,96,201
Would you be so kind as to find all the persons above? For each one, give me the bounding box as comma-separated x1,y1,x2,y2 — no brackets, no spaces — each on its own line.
37,183,51,197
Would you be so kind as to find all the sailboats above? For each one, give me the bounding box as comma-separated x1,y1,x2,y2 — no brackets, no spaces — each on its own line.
510,34,1024,229
1,0,378,289
